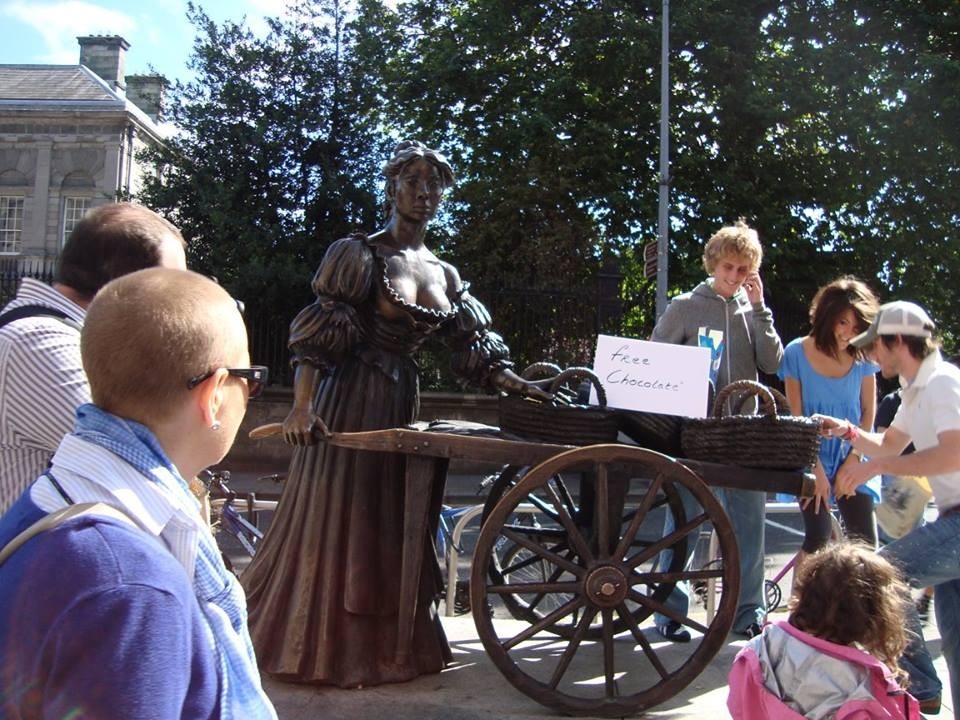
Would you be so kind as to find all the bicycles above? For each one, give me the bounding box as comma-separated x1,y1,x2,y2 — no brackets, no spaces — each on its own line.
201,468,288,581
434,461,591,627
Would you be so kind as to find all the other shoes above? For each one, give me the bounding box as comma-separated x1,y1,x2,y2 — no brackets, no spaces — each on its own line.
912,602,930,621
904,688,942,715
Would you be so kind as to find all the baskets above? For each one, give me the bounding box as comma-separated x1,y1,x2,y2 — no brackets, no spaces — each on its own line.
499,362,620,446
614,376,716,447
679,379,824,471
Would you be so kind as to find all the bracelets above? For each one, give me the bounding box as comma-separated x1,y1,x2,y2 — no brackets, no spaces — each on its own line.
850,448,868,463
840,422,859,443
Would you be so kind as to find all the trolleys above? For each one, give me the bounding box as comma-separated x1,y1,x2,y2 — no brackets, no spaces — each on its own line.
247,416,817,719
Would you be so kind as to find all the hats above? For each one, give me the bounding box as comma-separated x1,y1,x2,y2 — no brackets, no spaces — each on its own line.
848,300,935,348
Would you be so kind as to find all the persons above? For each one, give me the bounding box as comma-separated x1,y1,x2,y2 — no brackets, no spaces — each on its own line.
0,265,279,720
0,203,188,519
776,277,882,609
726,540,921,720
235,145,584,691
808,300,960,720
645,226,785,644
873,364,933,629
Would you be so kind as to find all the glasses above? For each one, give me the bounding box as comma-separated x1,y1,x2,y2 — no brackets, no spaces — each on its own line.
187,365,268,400
